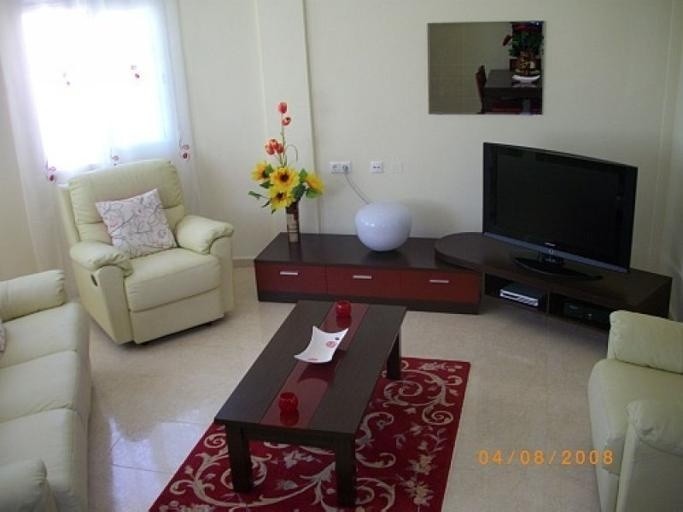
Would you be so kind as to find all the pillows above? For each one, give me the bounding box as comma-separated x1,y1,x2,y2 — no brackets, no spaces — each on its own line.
92,186,178,261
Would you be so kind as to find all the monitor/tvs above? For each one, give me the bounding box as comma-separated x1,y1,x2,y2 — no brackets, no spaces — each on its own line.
480,141,638,274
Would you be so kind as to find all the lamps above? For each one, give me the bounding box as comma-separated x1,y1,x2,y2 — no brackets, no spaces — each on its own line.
354,198,412,253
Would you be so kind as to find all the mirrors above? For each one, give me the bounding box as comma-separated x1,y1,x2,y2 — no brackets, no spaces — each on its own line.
425,19,545,118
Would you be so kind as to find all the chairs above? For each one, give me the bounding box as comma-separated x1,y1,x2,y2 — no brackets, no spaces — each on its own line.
588,305,682,512
475,62,523,114
55,156,235,348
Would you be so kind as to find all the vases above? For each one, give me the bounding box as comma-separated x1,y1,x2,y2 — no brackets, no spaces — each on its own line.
285,202,300,245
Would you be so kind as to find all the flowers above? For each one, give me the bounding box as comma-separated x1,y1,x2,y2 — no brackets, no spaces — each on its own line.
246,98,326,215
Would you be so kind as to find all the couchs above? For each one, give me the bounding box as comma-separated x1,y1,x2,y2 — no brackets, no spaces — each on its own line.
0,268,95,512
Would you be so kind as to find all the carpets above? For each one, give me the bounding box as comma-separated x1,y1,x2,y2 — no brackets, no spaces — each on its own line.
146,354,470,512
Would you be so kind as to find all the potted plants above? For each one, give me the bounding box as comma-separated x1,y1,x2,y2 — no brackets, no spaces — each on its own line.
501,20,544,83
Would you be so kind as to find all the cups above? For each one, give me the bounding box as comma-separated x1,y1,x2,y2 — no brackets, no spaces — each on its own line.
278,391,298,413
279,412,299,427
335,301,351,317
335,317,352,329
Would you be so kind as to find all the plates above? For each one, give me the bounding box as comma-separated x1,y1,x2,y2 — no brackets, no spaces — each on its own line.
295,349,350,386
292,325,349,365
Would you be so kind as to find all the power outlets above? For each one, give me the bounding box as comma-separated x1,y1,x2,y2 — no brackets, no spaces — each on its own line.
340,161,353,174
329,161,340,173
369,161,384,174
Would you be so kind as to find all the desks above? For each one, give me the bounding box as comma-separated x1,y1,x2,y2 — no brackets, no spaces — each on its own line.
211,298,410,509
480,67,542,114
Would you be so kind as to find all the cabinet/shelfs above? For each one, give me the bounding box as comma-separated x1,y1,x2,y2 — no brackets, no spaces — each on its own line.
432,231,675,334
250,231,485,315
